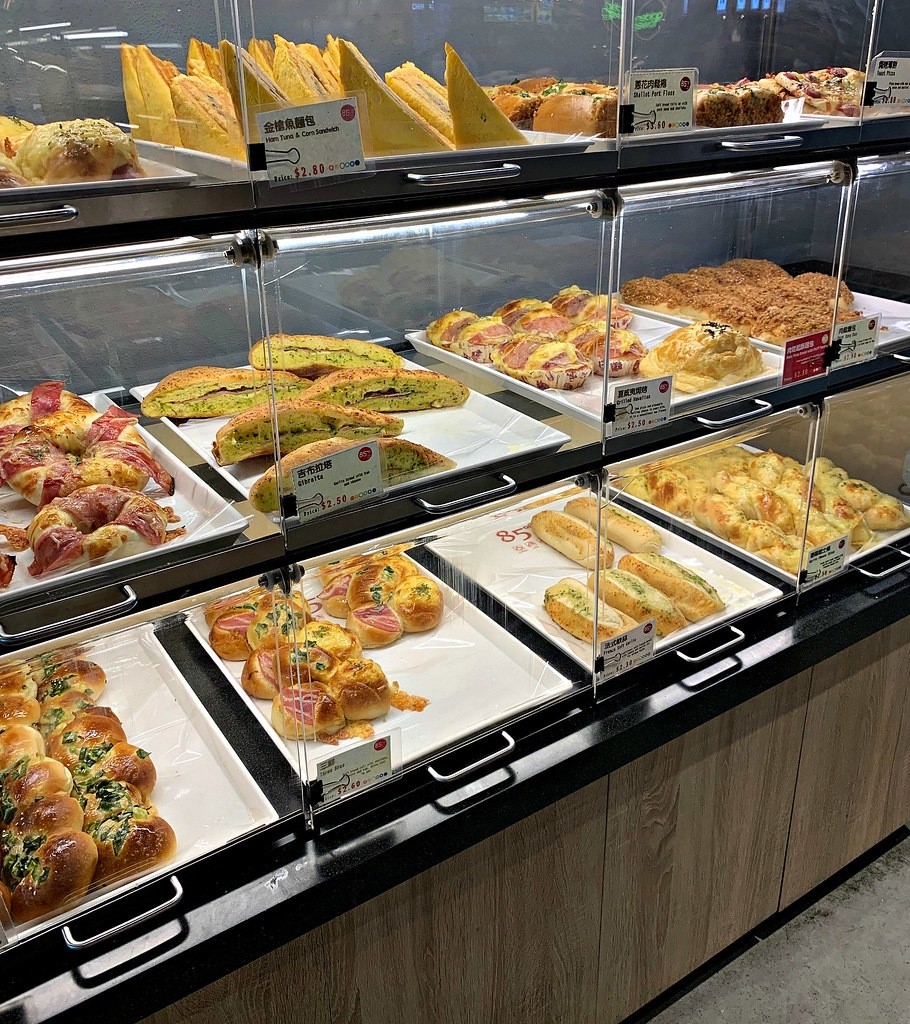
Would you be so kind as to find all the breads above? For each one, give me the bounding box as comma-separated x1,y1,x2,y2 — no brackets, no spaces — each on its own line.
0,34,910,923
